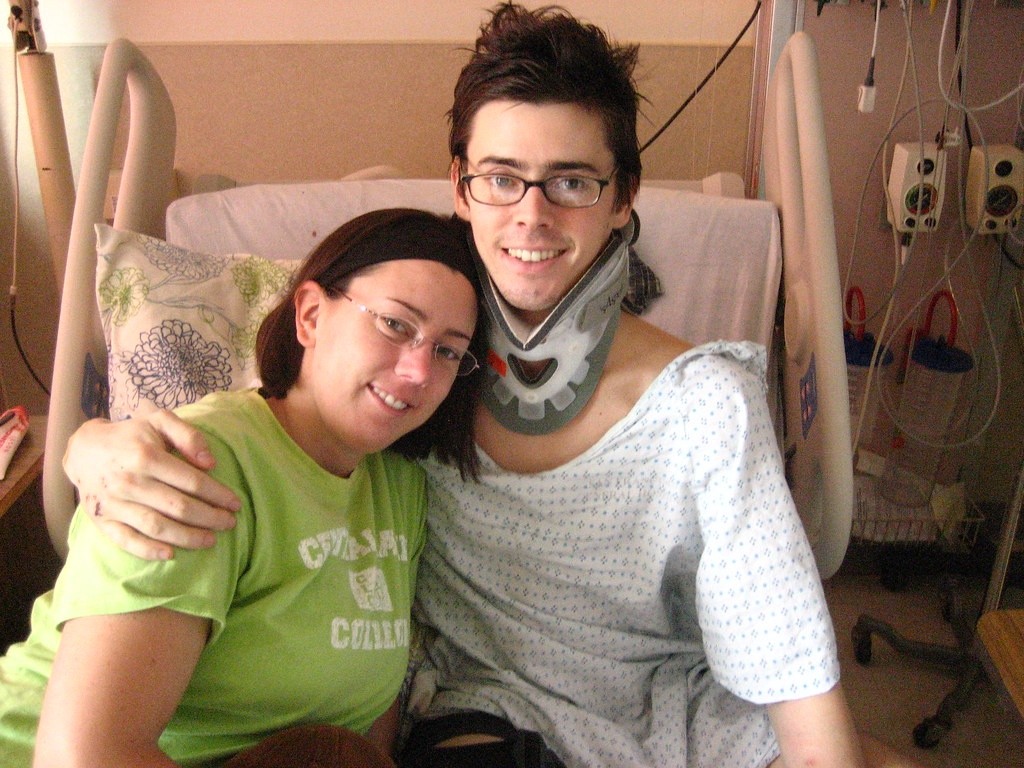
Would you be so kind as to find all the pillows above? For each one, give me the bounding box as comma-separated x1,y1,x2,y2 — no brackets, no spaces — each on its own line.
91,219,300,424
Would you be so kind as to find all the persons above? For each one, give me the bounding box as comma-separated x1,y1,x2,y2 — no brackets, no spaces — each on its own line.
59,0,871,768
0,207,488,768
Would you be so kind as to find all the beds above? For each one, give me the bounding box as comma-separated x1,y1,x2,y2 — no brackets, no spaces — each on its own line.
41,29,858,579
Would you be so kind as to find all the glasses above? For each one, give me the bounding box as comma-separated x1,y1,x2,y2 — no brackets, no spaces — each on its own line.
457,169,614,206
330,286,485,378
